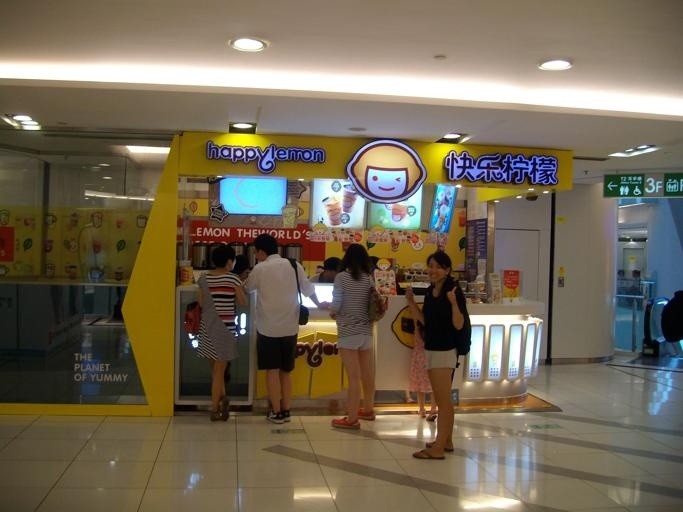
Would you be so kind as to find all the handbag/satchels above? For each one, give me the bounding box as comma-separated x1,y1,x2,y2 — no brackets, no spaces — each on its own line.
186,301,201,335
299,304,308,325
368,286,384,321
457,308,471,355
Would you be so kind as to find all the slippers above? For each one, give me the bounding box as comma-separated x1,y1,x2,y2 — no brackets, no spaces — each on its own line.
426,442,454,451
413,450,444,459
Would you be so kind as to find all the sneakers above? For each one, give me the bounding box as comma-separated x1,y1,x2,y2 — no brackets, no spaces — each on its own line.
210,396,437,429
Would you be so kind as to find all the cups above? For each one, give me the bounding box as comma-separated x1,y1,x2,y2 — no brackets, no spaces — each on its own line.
391,202,408,222
340,184,357,213
437,191,449,226
281,204,298,229
180,265,193,286
113,269,123,281
322,198,342,226
0,265,8,278
89,269,104,282
457,281,486,293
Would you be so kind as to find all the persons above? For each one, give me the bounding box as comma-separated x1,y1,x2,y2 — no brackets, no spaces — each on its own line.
308,256,345,284
242,233,331,425
196,246,247,422
328,243,377,432
404,251,466,460
403,285,439,421
